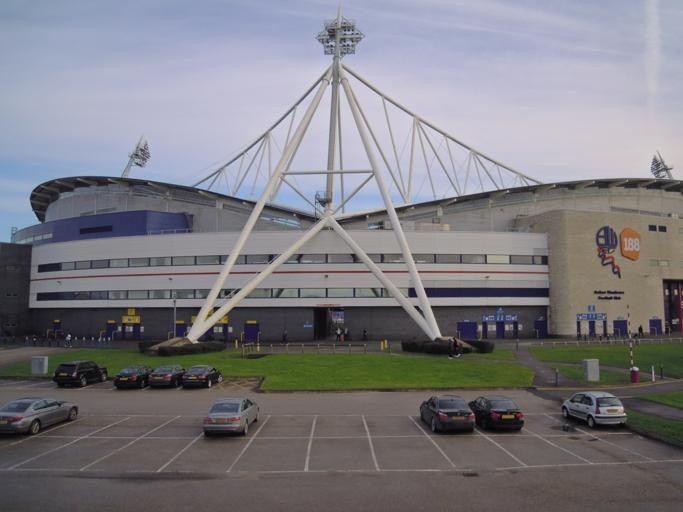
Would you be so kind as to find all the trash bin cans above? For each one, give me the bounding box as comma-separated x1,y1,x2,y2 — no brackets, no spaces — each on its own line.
629,366,640,383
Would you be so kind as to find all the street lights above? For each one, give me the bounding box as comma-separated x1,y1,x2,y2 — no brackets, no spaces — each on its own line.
484,275,490,339
168,275,176,339
55,279,65,338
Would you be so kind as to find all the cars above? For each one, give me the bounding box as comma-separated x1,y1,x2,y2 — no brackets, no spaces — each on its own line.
561,390,627,428
469,394,525,432
182,365,222,389
112,366,154,389
418,395,475,433
148,363,187,387
203,397,260,436
0,394,78,436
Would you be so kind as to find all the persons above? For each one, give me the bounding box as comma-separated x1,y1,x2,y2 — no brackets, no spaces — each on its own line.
448,337,459,354
64,331,72,348
335,327,343,342
638,325,644,336
362,328,369,341
279,326,289,346
24,334,40,345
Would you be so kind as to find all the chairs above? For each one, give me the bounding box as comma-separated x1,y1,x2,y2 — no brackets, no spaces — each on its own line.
240,320,262,352
100,320,118,342
46,319,63,340
168,320,186,340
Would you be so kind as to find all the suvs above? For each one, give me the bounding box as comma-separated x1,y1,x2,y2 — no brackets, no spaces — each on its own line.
52,359,108,388
